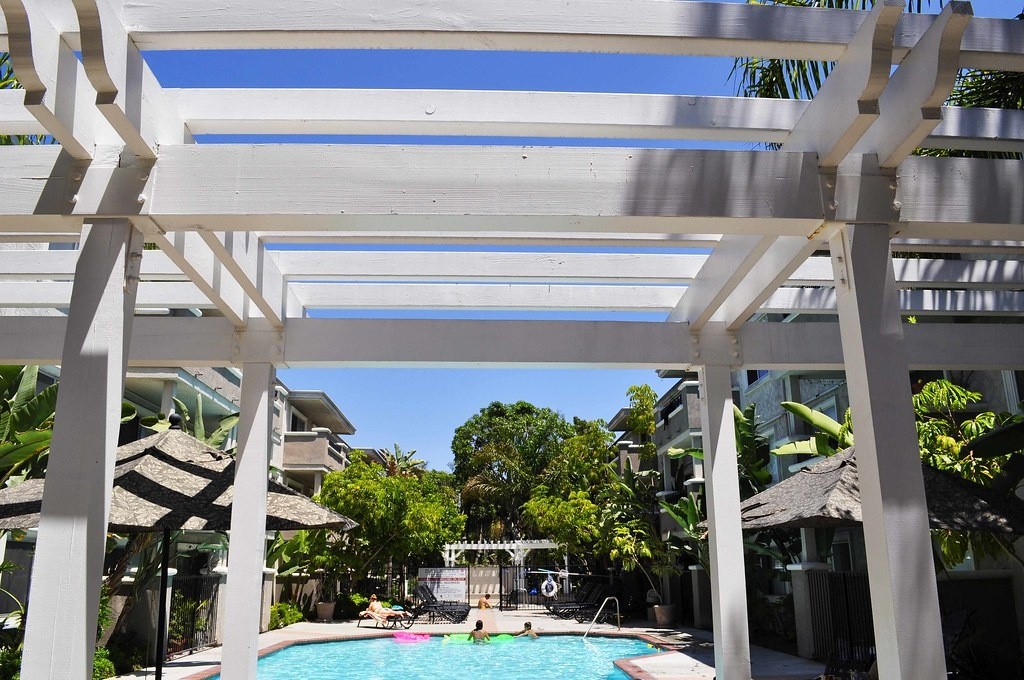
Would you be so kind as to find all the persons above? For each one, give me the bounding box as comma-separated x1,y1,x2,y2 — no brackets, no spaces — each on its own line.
468,621,490,644
478,594,493,610
366,594,414,618
514,622,538,640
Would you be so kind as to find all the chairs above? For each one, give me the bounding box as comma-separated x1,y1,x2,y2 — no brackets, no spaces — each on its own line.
357,583,471,629
501,589,518,609
543,577,627,623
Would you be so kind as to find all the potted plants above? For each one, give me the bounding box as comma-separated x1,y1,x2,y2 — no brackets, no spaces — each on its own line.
609,516,678,626
309,546,363,622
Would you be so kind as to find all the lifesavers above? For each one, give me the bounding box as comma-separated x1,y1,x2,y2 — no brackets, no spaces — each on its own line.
393,631,430,641
541,579,557,597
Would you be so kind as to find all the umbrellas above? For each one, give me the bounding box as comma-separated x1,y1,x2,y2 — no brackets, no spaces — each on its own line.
0,417,358,680
695,446,1024,531
